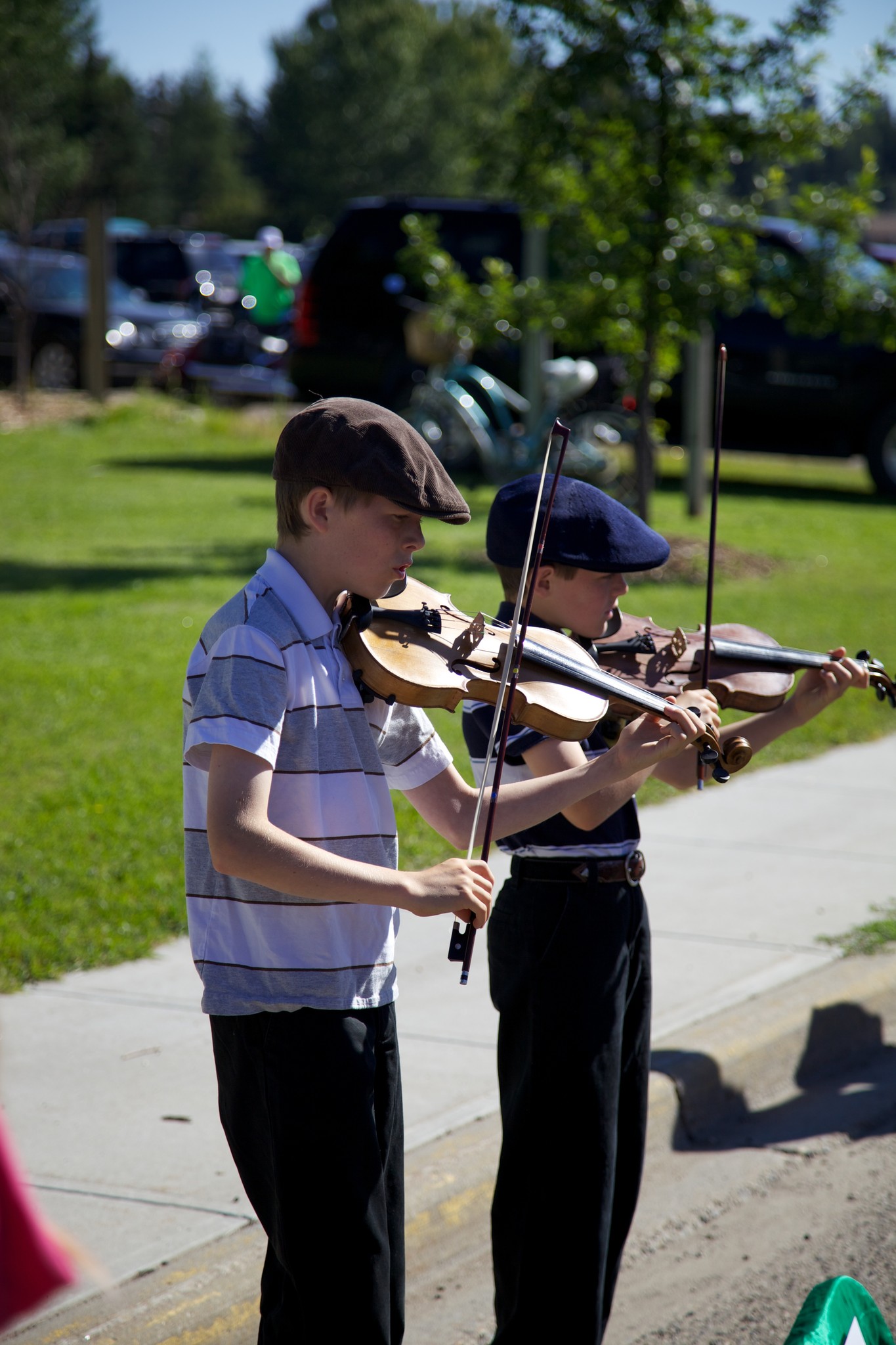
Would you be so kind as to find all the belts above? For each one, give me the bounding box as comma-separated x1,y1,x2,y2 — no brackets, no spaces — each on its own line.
509,850,646,888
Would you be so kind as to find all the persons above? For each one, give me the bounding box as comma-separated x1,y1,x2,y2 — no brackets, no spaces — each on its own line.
457,473,869,1345
180,398,708,1345
239,226,302,338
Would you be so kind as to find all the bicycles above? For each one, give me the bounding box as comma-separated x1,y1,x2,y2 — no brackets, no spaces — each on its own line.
390,291,653,515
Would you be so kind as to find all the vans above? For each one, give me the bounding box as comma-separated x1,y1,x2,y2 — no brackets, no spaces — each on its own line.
289,186,896,504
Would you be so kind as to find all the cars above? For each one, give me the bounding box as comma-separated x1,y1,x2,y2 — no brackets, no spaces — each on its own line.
0,216,302,406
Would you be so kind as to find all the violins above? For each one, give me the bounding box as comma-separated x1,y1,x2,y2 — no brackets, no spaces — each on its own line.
585,608,895,720
331,574,753,788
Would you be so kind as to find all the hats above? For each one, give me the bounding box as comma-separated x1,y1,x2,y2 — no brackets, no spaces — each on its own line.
270,396,471,524
486,473,671,573
